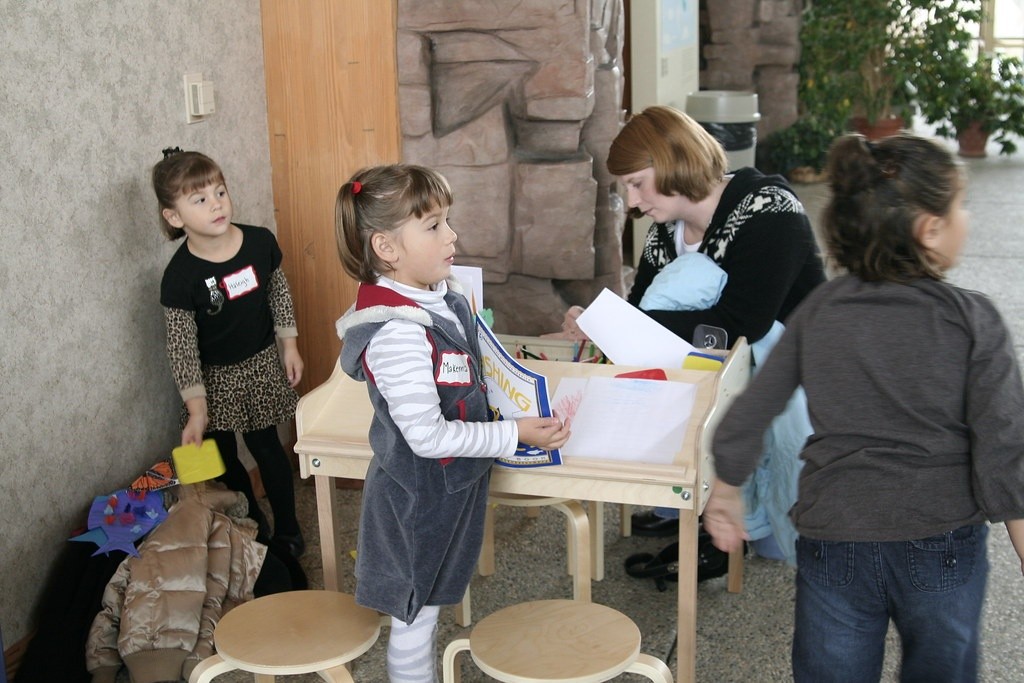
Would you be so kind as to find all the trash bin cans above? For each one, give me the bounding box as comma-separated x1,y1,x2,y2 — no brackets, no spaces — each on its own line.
686,89,760,173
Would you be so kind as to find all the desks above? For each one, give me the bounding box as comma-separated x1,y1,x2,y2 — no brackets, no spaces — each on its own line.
293,337,752,683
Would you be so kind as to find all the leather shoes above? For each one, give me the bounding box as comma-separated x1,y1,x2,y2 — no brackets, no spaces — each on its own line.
631,510,703,537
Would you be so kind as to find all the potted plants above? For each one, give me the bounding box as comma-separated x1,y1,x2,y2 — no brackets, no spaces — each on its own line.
761,0,1024,185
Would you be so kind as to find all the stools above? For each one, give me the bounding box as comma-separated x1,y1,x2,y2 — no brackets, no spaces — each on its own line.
189,589,380,683
442,599,675,683
477,492,591,603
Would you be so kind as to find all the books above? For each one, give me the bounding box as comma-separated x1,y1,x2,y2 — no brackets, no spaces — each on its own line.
476,312,563,468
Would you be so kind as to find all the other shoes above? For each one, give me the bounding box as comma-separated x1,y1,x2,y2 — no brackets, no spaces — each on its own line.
253,564,274,599
272,560,308,592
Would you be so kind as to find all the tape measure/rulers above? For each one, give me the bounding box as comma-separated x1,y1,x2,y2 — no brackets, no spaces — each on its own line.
727,520,743,594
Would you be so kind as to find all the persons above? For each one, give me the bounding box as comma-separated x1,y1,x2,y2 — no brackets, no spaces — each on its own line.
564,107,826,584
333,164,571,682
705,134,1024,683
151,147,304,556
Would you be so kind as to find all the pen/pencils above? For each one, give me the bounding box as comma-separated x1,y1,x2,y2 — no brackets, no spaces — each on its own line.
665,634,677,665
589,344,595,363
516,344,521,359
573,339,579,362
594,354,612,364
521,348,543,360
522,345,527,359
471,289,476,315
539,353,548,360
578,339,586,359
